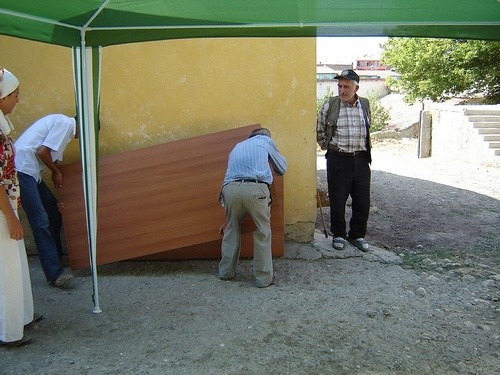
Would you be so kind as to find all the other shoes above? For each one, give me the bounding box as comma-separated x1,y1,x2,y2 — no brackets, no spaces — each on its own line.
34,313,42,322
0,336,33,347
54,273,73,286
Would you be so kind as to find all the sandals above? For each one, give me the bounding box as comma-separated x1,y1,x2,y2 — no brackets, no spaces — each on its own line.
347,235,369,252
332,237,346,250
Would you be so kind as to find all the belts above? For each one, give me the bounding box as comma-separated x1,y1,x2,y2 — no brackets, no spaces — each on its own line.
234,179,264,183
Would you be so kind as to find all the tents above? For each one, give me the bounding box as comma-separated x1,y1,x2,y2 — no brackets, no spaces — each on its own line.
0,0,500,313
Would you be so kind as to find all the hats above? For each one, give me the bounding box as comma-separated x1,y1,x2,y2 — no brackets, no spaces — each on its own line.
334,69,360,83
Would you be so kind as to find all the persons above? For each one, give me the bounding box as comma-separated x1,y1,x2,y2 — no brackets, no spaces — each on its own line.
219,127,287,287
15,114,100,288
317,68,373,252
0,68,44,346
